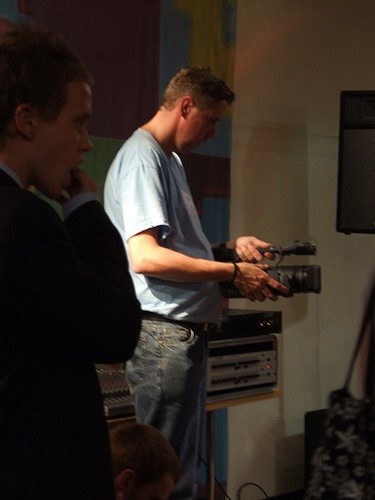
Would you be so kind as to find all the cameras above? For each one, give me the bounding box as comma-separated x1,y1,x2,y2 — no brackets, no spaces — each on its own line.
210,243,321,298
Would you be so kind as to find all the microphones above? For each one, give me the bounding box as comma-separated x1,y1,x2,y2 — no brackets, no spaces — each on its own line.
256,242,317,256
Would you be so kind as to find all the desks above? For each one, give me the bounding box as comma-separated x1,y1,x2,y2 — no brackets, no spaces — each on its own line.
106,388,284,500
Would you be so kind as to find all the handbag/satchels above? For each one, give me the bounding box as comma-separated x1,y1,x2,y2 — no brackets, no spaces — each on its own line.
307,387,375,500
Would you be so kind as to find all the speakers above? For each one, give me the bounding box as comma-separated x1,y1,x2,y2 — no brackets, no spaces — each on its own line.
334,90,375,234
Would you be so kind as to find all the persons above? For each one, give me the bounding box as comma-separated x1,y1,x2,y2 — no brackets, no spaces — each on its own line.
103,65,292,500
0,26,142,500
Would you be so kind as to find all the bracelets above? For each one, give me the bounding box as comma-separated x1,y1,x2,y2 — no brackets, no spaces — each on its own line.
228,261,238,282
